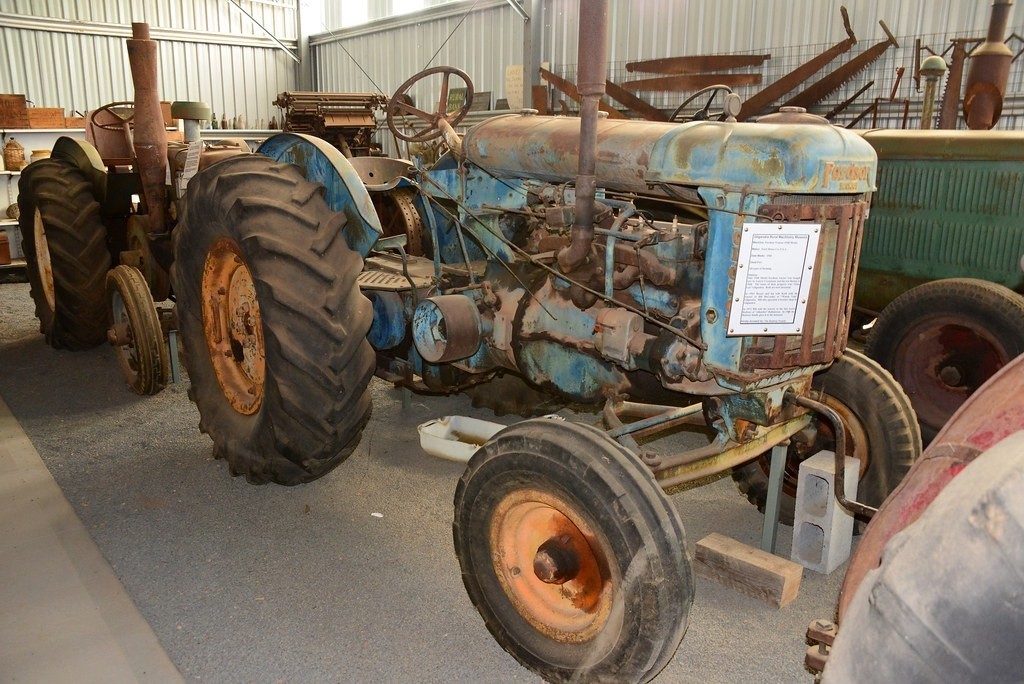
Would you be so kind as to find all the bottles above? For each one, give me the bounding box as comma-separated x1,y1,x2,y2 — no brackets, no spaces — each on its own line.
281,116,285,129
221,112,228,129
212,113,218,129
272,116,277,129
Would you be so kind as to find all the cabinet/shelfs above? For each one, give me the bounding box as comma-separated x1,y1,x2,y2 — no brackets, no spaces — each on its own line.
0,129,283,268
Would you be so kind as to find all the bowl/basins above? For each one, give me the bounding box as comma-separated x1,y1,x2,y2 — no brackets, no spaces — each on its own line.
417,414,508,463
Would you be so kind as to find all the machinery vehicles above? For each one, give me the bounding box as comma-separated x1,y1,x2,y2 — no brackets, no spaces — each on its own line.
168,2,926,683
19,24,269,396
666,2,1024,450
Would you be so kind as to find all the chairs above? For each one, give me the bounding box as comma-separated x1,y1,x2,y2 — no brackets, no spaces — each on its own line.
345,154,414,192
85,109,135,165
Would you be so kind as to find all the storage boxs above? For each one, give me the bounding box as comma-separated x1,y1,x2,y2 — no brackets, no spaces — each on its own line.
0,94,29,129
65,117,85,128
28,108,66,129
0,229,11,265
